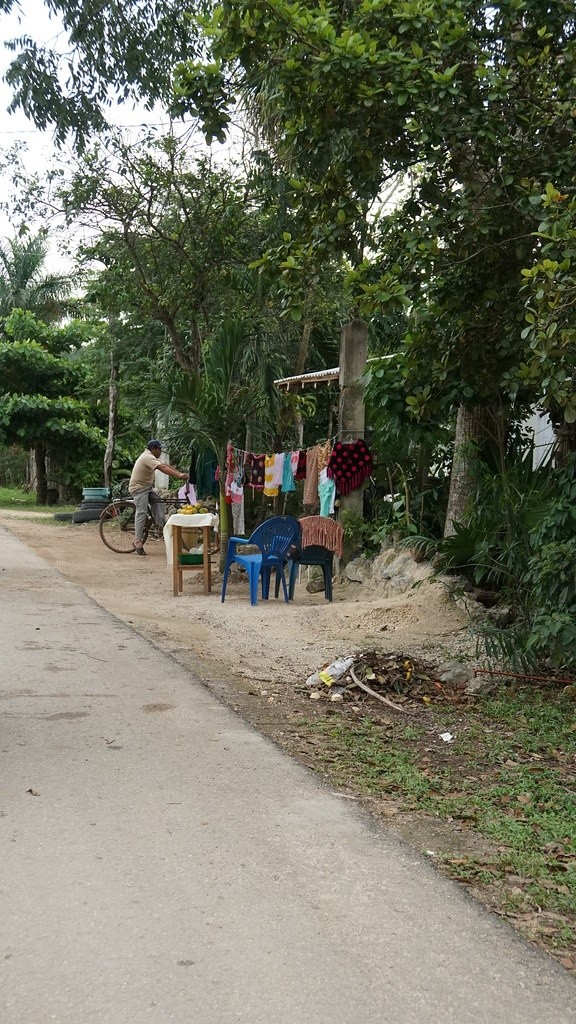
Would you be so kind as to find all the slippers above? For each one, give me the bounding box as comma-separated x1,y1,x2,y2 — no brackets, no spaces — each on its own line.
132,542,147,555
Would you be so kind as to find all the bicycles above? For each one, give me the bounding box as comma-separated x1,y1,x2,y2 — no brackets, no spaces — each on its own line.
98,478,222,555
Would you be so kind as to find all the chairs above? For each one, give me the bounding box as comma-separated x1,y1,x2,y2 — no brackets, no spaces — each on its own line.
221,515,301,606
275,515,345,603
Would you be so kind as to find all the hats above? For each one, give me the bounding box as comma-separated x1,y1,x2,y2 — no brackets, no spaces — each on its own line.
147,439,165,448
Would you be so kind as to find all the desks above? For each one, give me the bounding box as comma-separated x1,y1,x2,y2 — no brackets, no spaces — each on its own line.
172,513,220,598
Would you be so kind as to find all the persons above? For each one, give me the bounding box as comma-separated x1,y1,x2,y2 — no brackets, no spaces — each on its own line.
128,440,188,555
